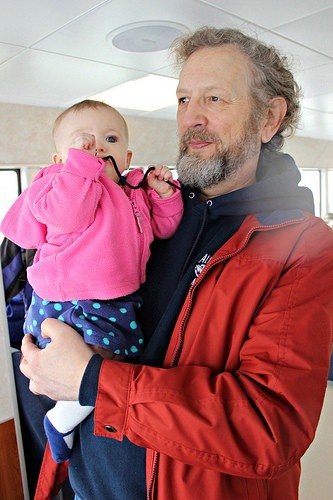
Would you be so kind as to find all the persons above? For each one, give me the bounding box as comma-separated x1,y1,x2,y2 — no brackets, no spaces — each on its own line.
0,99,183,463
19,28,333,500
